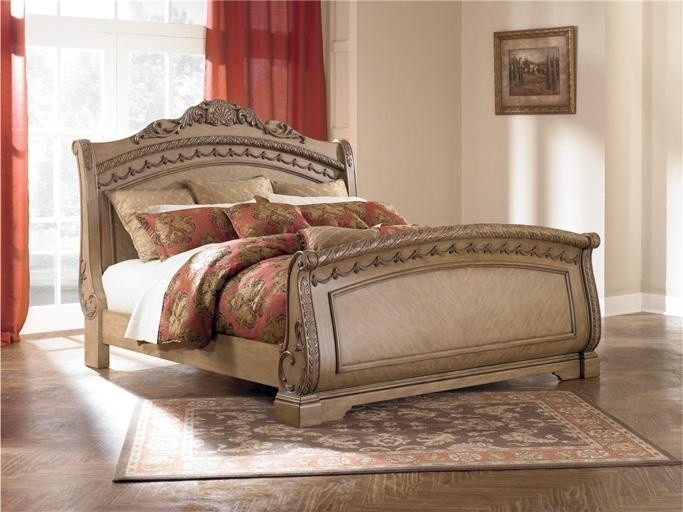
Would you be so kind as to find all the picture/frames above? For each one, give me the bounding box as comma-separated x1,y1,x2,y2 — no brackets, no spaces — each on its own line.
493,25,576,116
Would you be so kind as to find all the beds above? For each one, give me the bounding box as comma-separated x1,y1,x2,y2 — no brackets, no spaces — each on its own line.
70,97,605,430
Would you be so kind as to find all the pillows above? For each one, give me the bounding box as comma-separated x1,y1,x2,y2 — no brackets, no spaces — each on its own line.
177,174,274,204
222,202,312,239
254,192,367,203
135,207,240,264
103,186,196,263
270,178,348,197
295,201,407,228
145,198,258,214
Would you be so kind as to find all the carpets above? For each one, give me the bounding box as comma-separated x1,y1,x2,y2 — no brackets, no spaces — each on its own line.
112,385,681,494
